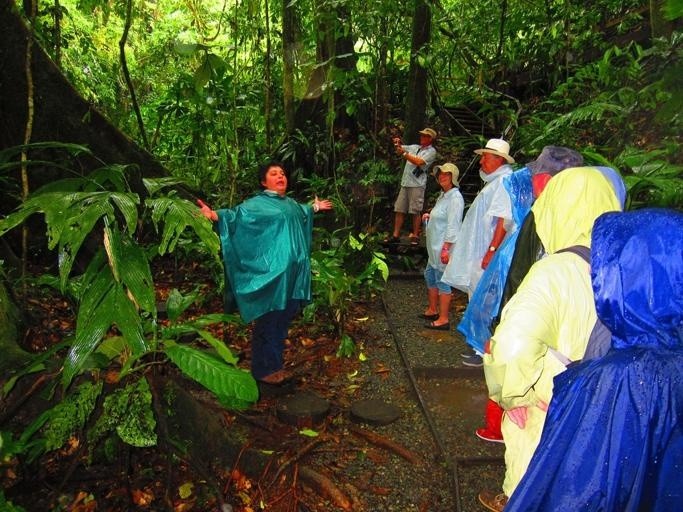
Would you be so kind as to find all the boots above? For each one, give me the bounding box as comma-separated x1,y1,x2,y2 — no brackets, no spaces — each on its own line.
476,400,506,443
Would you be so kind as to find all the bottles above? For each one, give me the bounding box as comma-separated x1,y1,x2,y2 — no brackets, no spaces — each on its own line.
422,216,429,238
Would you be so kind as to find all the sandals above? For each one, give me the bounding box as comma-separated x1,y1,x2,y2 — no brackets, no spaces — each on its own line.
410,237,420,245
384,235,400,243
418,312,439,320
424,321,449,330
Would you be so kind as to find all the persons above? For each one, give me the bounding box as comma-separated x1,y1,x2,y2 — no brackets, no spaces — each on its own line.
460,145,582,354
503,209,683,511
195,161,333,387
441,139,517,367
480,166,624,511
458,168,535,353
417,162,465,330
378,128,437,245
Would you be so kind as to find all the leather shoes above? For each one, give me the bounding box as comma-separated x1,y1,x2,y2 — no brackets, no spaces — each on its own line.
479,489,508,512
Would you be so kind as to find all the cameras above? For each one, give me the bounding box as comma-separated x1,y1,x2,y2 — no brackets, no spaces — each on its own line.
393,141,400,149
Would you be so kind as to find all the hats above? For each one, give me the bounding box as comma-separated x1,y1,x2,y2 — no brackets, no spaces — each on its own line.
525,146,582,177
474,138,515,164
419,128,436,139
432,162,459,188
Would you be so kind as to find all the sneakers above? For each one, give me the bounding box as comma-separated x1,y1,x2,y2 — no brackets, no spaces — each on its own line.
463,356,483,367
258,371,288,384
278,368,296,378
461,351,474,357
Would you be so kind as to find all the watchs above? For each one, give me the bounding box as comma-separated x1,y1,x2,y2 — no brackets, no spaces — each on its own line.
488,246,496,252
401,151,408,158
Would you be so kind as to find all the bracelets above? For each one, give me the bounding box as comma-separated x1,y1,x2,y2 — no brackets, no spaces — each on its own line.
443,247,449,250
313,204,319,213
